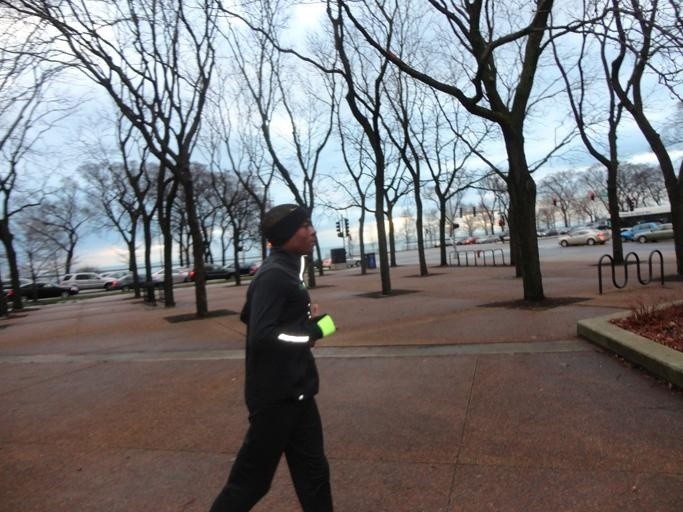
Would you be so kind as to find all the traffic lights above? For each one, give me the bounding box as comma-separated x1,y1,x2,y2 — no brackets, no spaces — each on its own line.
591,192,594,201
459,207,462,219
553,198,557,207
345,218,350,237
336,220,341,233
472,206,477,217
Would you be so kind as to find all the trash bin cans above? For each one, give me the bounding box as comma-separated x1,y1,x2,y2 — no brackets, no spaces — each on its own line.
364,253,376,269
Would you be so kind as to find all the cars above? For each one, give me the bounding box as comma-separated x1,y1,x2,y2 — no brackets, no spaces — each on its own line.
556,203,674,246
433,226,556,248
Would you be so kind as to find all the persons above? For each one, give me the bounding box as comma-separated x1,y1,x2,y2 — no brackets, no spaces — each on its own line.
209,202,338,511
